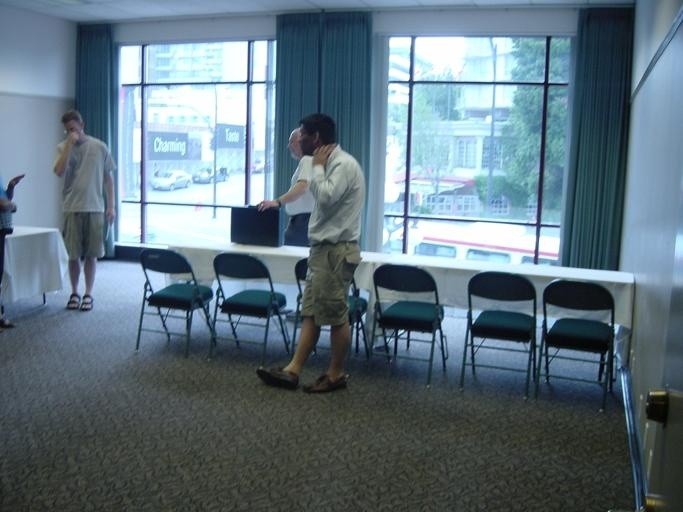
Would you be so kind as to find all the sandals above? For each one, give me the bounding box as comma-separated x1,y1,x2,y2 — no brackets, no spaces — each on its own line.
68,294,80,308
81,295,93,310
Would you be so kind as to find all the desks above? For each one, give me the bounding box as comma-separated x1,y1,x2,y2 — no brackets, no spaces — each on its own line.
164,242,636,394
0,224,72,309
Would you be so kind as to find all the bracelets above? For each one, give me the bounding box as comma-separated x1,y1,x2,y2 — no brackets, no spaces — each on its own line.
276,198,282,208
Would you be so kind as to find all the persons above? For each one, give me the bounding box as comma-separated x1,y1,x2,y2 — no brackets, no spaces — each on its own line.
256,113,366,394
0,173,24,327
51,110,116,312
256,127,323,246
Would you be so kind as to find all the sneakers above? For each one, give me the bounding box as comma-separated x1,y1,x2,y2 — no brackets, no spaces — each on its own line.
257,366,298,388
304,374,346,392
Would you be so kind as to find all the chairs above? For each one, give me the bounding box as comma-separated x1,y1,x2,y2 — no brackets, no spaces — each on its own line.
290,257,368,361
368,262,448,390
457,270,537,404
134,248,215,360
534,277,616,415
209,251,291,371
